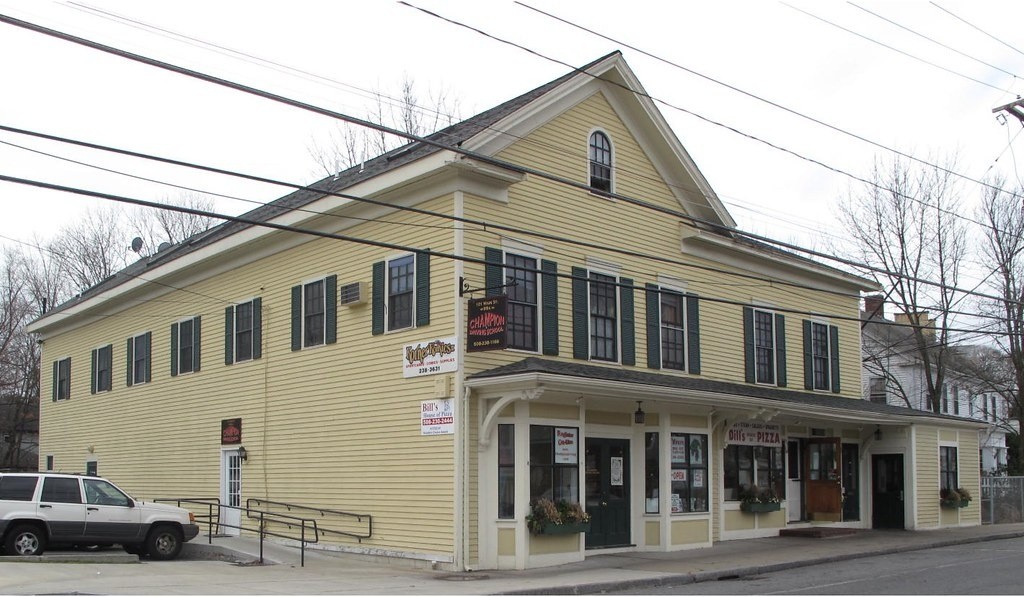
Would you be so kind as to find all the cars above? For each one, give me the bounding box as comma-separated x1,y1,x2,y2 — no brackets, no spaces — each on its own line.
1,473,200,561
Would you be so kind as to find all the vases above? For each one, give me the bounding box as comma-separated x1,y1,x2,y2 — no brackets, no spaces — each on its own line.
540,522,588,535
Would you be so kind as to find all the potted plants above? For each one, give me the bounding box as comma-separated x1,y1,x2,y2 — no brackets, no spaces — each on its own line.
741,486,780,513
942,488,972,509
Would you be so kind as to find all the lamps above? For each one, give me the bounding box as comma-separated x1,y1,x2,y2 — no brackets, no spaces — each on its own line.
635,400,644,424
237,446,247,461
36,339,45,345
874,425,882,441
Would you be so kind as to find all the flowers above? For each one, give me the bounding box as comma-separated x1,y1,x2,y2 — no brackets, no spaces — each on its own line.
525,497,589,537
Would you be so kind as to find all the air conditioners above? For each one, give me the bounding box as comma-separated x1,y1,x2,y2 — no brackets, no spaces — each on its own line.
340,282,368,307
